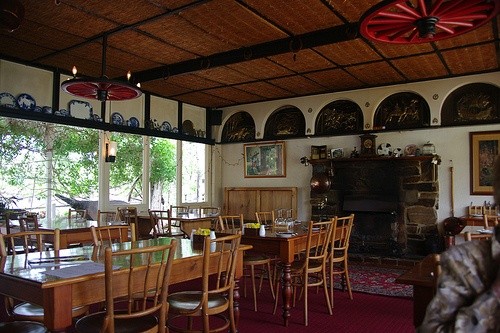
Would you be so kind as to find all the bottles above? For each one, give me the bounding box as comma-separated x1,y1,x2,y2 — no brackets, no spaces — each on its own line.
190,228,216,253
259,225,265,237
275,217,296,229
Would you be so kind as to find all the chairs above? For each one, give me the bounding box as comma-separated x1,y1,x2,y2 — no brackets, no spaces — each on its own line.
0,206,355,333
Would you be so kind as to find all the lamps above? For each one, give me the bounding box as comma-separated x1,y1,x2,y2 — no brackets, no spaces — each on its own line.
106,141,117,162
62,36,143,101
358,0,500,43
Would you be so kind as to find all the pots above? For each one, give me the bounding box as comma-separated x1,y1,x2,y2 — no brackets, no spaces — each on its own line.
309,172,331,193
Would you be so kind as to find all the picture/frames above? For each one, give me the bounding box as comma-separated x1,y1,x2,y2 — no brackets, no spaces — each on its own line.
469,130,500,195
244,142,285,177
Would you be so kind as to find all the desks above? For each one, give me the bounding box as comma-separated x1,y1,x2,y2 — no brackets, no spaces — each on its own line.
215,223,353,327
396,254,437,326
36,217,129,250
0,236,252,333
127,214,218,234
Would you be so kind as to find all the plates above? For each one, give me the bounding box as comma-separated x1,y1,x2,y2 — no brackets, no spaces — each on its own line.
0,91,195,134
305,227,320,231
394,147,403,157
404,144,417,155
471,214,484,216
476,229,493,234
276,232,292,236
377,142,393,156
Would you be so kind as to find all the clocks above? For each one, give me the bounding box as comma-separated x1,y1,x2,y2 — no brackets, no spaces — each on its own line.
359,135,376,154
